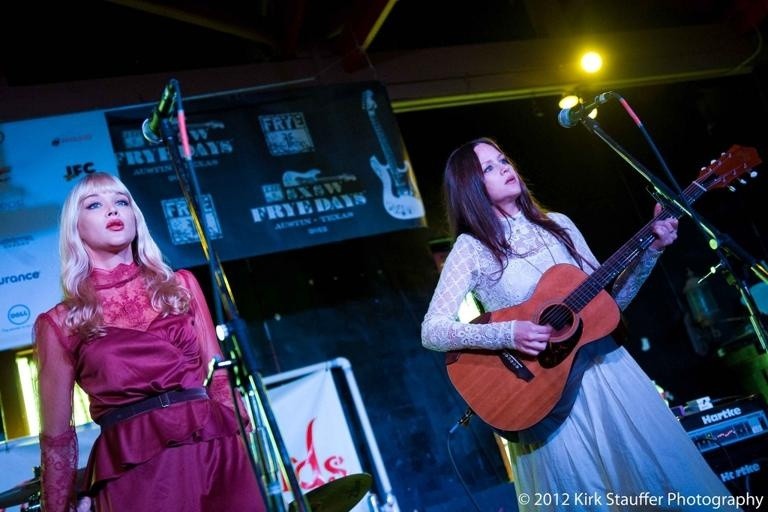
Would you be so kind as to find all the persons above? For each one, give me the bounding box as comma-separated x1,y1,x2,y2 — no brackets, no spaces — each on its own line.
32,170,274,512
420,137,744,511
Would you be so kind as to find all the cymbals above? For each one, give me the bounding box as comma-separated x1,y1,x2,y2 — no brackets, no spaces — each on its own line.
0,467,90,508
289,472,373,512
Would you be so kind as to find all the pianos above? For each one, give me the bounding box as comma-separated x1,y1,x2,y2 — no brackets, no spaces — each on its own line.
676,395,767,453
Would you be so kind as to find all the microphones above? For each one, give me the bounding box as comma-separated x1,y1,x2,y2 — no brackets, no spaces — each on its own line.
140,84,177,146
558,90,612,129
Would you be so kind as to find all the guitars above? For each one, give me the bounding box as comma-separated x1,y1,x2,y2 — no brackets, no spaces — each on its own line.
445,144,762,444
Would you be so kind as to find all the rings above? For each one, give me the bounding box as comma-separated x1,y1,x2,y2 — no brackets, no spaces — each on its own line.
668,228,674,234
